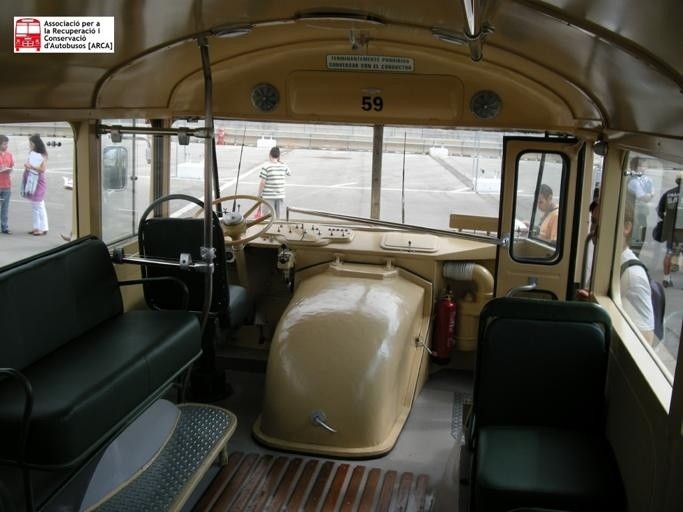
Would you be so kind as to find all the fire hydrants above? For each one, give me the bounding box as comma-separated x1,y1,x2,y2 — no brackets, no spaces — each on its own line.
217,128,225,144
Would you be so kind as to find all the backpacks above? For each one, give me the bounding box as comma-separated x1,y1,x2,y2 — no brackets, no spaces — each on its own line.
620,259,666,345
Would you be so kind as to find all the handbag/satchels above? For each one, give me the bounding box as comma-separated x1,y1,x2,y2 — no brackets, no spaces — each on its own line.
652,220,668,243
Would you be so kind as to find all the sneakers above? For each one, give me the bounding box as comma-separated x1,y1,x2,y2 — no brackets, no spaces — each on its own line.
3,229,72,242
662,280,673,288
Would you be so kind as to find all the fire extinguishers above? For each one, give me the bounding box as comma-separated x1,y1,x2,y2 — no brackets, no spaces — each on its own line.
435,287,457,363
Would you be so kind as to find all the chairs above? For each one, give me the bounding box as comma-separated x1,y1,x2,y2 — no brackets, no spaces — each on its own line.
139,195,250,337
465,298,615,507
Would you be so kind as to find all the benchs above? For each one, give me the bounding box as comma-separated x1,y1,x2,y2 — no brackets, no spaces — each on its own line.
1,234,207,509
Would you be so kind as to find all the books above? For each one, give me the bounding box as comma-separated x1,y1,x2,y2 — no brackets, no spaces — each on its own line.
62,177,74,190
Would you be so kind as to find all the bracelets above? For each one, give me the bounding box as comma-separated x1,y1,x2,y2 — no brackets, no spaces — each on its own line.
257,192,263,195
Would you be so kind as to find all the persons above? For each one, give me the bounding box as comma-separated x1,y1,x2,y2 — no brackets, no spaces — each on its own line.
522,183,559,244
627,156,655,259
593,164,602,188
657,175,683,287
0,135,13,235
61,187,74,241
22,134,49,235
576,197,655,345
256,147,291,219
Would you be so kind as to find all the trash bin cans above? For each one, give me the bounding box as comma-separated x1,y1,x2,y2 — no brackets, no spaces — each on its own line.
218,128,225,145
